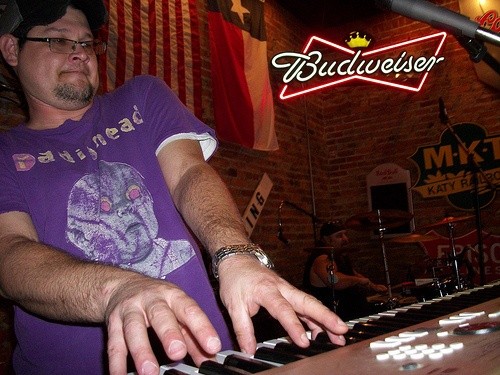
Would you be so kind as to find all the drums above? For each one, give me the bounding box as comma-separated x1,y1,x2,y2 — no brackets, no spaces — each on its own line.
429,277,468,298
364,293,420,316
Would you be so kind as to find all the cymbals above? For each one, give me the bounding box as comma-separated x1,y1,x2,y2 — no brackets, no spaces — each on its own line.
302,246,359,255
346,211,414,231
423,215,475,228
388,233,439,243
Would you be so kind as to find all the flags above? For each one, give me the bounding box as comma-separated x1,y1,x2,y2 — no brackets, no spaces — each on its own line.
205,0,279,152
101,1,202,123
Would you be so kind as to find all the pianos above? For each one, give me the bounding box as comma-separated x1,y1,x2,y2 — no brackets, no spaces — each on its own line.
129,280,500,375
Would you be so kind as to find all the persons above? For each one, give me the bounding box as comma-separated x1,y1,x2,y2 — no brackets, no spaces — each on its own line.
0,0,350,375
305,222,387,323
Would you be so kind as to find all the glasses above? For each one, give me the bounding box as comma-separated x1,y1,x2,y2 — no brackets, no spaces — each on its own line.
20,36,107,55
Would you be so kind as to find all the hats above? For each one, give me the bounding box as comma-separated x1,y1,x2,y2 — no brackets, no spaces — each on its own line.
2,0,106,34
321,219,345,236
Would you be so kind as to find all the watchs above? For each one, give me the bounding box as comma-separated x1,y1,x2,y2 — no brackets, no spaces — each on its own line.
212,244,274,281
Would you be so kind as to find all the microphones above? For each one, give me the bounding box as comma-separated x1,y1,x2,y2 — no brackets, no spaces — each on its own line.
438,99,449,123
276,234,292,245
